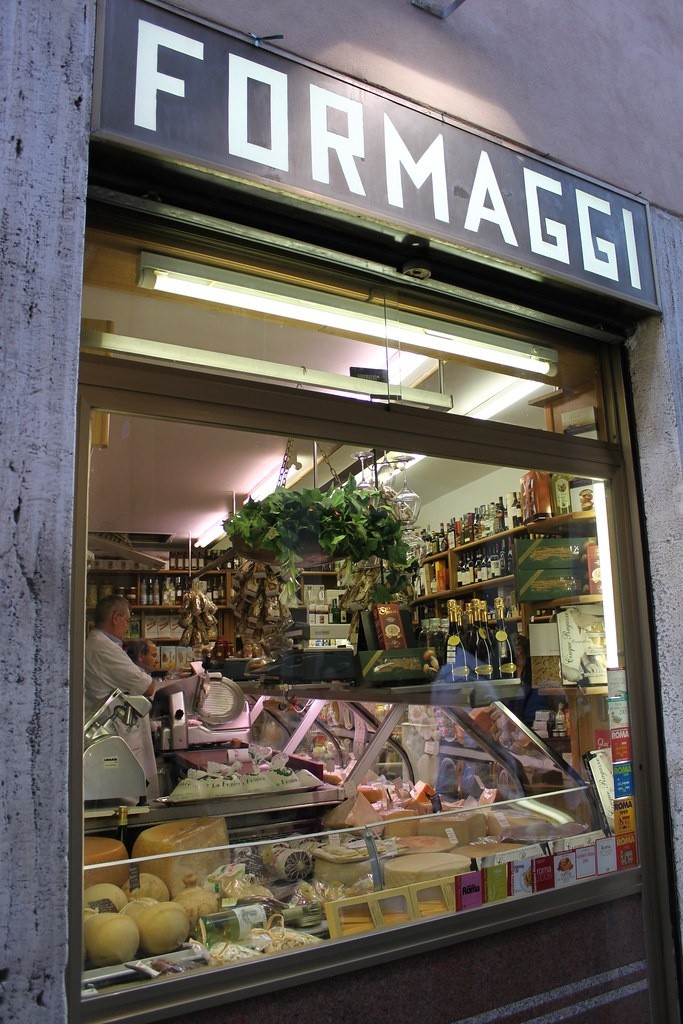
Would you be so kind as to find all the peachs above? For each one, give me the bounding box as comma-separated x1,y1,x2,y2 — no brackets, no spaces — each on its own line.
83,873,189,963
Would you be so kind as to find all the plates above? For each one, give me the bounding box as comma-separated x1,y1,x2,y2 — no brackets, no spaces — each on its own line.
520,869,532,891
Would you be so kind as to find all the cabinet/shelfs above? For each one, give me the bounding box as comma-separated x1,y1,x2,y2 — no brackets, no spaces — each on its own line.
410,510,603,626
86,568,239,672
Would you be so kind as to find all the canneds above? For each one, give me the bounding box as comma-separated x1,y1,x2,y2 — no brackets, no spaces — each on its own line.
117,587,126,598
126,587,137,605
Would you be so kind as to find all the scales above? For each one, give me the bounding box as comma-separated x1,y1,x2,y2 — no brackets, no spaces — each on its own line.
84,688,153,807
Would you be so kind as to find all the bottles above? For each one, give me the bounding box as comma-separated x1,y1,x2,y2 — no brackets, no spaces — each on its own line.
411,491,555,597
195,906,303,950
235,627,243,658
329,595,350,623
142,576,225,606
319,586,325,604
412,598,516,683
171,551,204,570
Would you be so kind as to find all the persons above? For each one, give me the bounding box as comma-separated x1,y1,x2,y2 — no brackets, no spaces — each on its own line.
435,620,517,800
126,638,158,675
84,594,161,803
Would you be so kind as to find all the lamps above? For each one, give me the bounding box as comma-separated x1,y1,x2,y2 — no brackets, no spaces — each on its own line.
132,251,559,379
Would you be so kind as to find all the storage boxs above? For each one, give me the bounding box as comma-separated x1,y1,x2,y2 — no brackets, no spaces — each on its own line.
373,604,408,650
143,615,201,670
513,538,598,569
353,647,438,688
514,568,589,603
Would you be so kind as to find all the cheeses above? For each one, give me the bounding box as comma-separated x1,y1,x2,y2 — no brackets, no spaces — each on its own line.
314,774,587,896
140,818,233,895
84,836,131,886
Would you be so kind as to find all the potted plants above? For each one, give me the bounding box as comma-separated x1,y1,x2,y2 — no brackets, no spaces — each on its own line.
223,481,423,608
